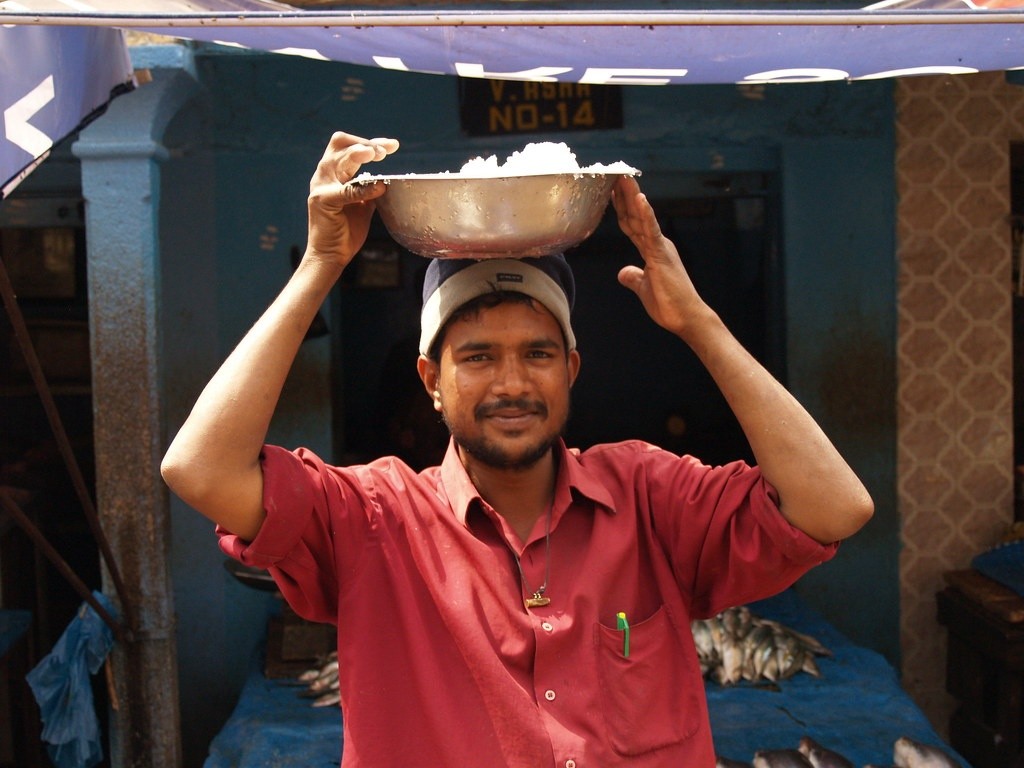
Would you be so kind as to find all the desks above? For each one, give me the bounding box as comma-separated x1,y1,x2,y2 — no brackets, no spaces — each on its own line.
934,586,1024,768
200,626,947,768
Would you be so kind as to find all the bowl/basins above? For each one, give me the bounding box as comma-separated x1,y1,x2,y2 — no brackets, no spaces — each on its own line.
350,169,640,257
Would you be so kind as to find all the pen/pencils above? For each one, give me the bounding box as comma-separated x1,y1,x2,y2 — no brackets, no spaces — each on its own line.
615,611,630,659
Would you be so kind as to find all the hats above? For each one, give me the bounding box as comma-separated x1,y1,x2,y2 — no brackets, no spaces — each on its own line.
419,253,577,358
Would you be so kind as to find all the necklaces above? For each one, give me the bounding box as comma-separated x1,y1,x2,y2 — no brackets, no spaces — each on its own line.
503,457,558,608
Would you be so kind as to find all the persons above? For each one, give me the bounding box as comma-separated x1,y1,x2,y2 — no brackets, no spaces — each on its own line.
159,131,875,768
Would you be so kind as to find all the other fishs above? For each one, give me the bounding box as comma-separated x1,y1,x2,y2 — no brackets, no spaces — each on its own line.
712,735,965,768
691,602,836,690
300,647,343,712
